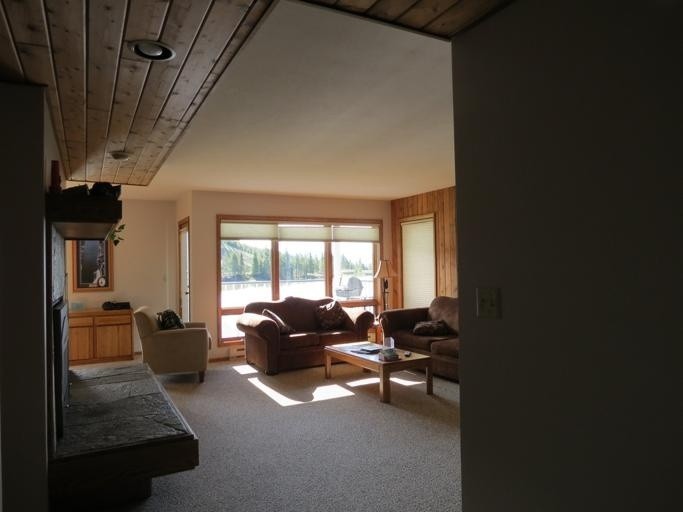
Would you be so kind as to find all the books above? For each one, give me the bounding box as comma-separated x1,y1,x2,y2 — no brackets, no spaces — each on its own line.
350,343,382,354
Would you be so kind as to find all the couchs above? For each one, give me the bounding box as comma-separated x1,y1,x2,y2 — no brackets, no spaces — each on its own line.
376,293,458,380
234,297,373,377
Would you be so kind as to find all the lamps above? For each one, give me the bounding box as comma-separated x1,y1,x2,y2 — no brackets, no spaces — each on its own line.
372,259,397,309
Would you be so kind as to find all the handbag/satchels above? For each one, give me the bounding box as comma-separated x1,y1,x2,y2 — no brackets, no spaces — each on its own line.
161,309,185,329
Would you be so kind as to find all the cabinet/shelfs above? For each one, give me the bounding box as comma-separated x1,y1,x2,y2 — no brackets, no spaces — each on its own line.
67,303,132,364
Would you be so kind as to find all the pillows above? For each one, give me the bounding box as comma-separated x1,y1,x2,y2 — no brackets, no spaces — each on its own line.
260,307,297,335
412,316,447,335
313,299,344,327
157,309,187,330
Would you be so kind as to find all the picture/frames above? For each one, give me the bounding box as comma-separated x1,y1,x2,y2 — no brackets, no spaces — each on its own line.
70,238,114,291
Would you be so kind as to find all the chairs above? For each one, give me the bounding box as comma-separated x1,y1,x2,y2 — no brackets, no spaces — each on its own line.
134,304,209,383
